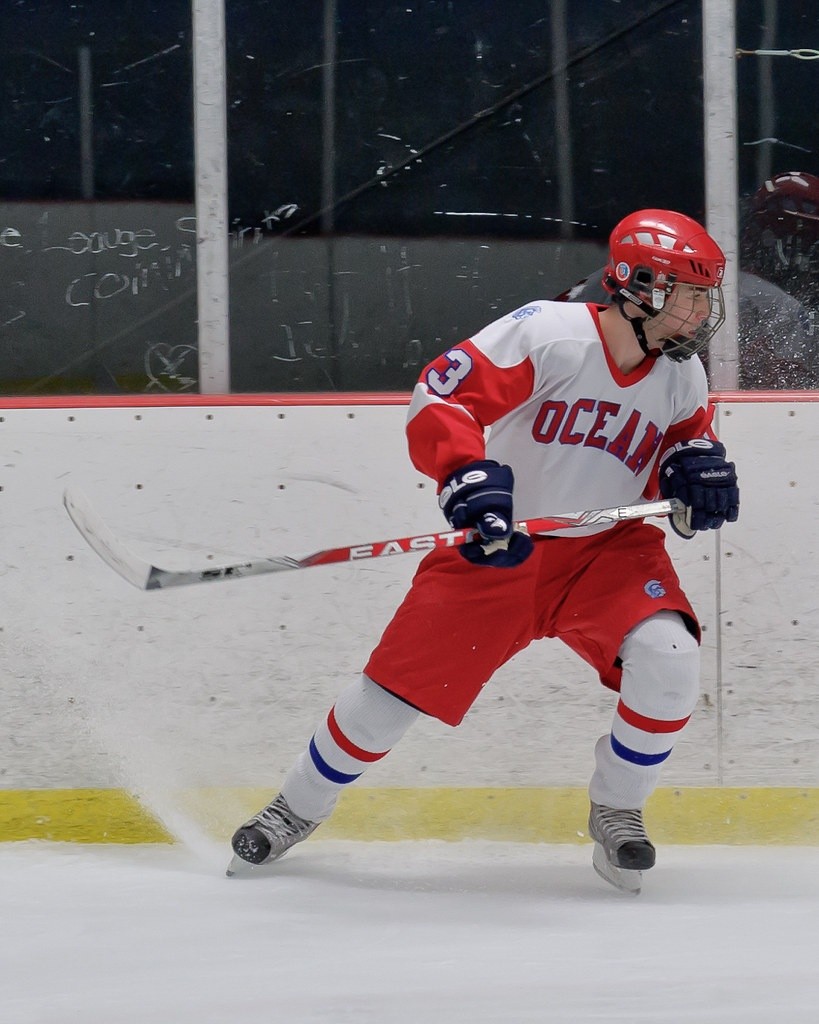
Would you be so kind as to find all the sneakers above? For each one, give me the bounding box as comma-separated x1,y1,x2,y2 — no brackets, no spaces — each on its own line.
226,793,321,877
588,798,657,895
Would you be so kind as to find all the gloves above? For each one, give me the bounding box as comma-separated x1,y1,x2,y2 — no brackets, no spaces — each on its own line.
439,459,535,567
658,438,740,539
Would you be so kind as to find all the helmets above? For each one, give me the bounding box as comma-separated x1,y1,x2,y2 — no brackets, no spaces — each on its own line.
600,209,727,363
752,172,819,241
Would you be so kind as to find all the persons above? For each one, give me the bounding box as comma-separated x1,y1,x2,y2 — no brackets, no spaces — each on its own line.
227,210,740,895
738,171,819,391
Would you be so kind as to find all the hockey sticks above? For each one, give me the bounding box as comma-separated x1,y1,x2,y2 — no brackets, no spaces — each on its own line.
63,484,683,592
